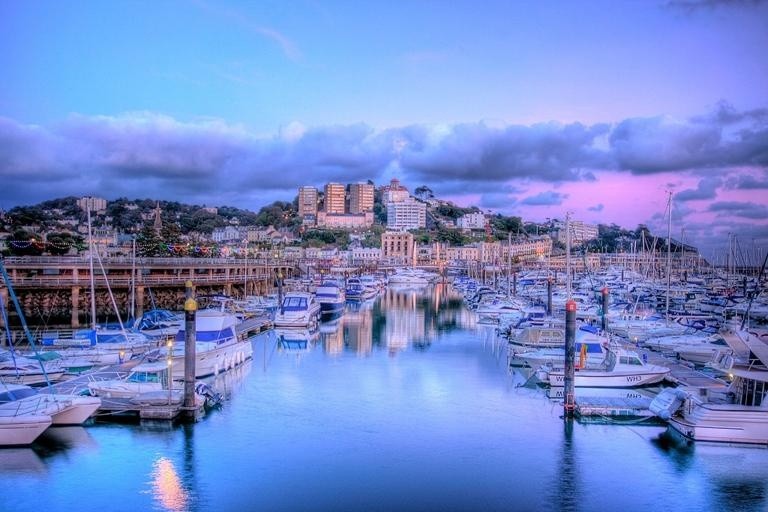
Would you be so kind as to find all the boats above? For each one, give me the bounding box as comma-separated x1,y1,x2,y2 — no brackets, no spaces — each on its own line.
573,412,768,457
1,427,100,472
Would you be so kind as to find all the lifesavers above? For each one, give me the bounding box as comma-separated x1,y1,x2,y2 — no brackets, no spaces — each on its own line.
345,272,349,278
581,345,587,367
